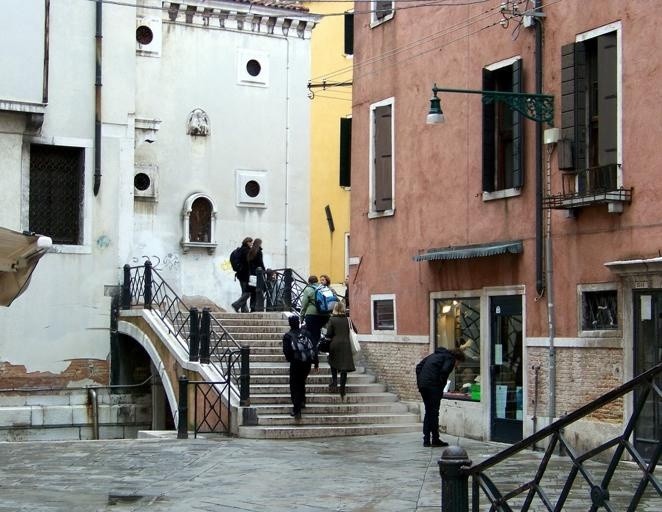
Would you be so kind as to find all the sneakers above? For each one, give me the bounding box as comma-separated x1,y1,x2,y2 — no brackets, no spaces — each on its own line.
291,412,301,418
424,440,448,447
301,402,305,408
232,304,265,312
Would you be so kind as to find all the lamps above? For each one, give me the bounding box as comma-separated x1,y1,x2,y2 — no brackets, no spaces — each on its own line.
426,83,555,128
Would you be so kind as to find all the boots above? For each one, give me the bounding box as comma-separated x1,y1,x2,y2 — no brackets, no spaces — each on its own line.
329,367,337,387
340,372,347,395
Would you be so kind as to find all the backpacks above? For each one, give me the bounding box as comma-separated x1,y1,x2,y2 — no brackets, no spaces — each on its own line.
287,329,315,364
308,284,336,313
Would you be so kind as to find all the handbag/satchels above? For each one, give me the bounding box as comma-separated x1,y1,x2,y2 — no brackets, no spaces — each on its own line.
317,338,332,352
230,247,244,271
349,328,361,355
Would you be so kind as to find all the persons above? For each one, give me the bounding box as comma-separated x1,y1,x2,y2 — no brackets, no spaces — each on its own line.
319,275,337,298
413,346,466,448
323,301,357,396
245,237,267,311
231,236,254,313
282,315,320,419
343,274,350,317
298,275,328,364
